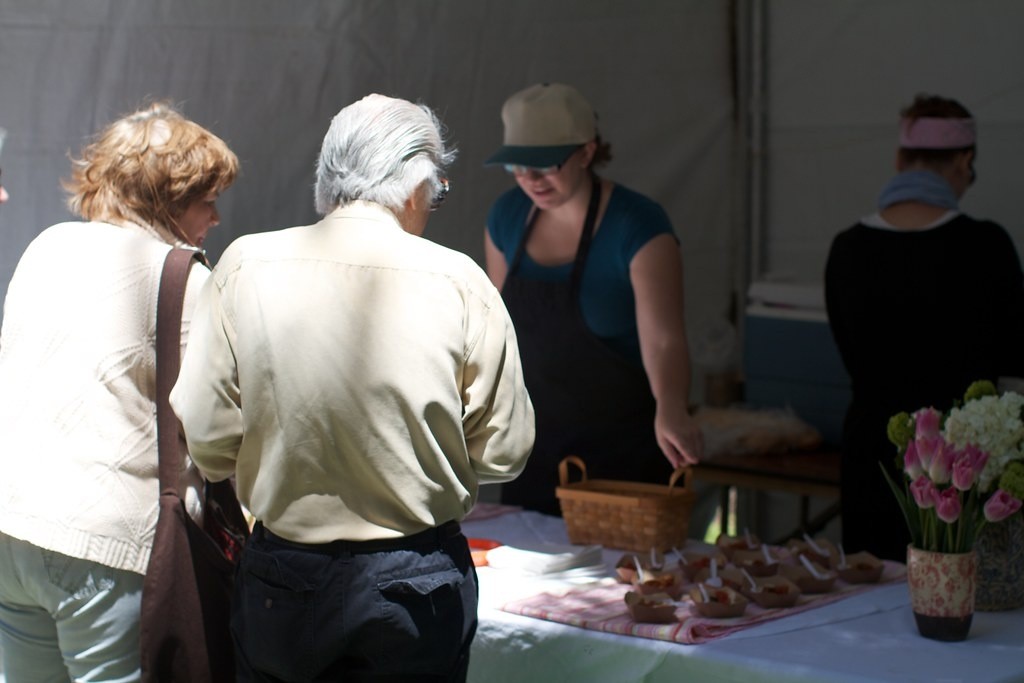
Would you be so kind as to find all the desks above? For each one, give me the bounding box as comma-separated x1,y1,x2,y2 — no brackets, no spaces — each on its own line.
460,504,1024,683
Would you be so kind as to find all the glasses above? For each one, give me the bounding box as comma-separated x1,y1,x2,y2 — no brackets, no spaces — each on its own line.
430,172,449,212
504,160,567,176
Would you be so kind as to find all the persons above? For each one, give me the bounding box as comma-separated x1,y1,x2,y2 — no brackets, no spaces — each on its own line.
168,93,534,682
823,93,1024,563
1,103,238,683
486,82,702,517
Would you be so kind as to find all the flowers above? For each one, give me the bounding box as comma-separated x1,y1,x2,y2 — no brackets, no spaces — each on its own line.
883,378,1024,549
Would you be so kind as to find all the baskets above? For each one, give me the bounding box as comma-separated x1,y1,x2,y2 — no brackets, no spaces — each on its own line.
556,456,694,553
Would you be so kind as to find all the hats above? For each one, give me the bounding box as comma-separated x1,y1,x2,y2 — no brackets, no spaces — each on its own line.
482,81,598,168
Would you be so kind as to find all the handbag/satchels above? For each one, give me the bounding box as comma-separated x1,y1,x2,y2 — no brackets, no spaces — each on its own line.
139,474,251,683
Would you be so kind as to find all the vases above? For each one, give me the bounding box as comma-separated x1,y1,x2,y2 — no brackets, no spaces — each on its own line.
909,545,984,641
